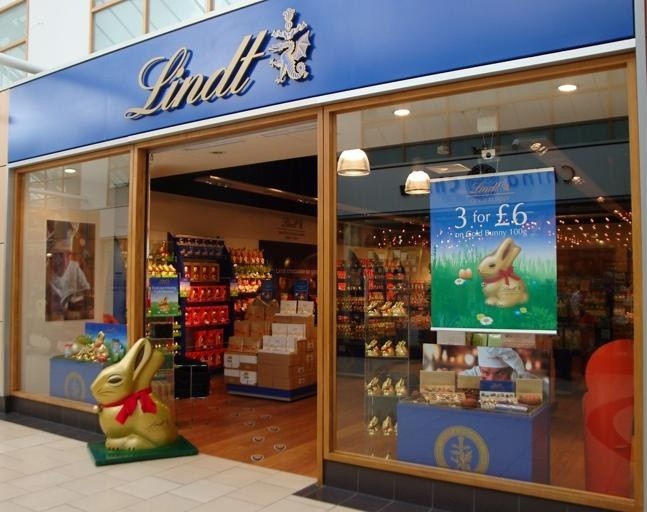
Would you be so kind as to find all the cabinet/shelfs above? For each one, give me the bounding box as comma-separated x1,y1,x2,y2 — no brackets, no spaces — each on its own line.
144,232,234,379
227,241,318,302
336,249,437,459
558,272,633,368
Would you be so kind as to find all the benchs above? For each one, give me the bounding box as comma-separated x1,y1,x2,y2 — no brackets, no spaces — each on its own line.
174,356,210,399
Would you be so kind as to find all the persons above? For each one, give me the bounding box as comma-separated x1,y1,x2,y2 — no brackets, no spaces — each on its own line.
46,249,91,319
435,346,548,397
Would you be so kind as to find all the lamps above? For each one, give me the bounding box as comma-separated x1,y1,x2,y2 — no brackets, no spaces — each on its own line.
404,157,431,195
337,149,370,176
437,145,449,155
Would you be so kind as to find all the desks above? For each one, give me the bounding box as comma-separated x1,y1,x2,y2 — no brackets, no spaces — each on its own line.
395,397,555,485
49,355,108,405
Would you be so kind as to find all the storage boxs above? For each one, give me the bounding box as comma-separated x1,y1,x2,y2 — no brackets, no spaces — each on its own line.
225,301,317,391
